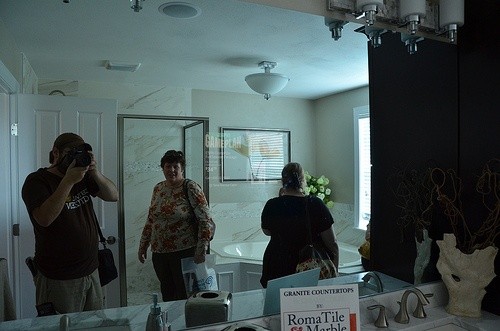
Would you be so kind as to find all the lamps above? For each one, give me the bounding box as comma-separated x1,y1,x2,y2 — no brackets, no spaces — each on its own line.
400,0,426,35
357,0,383,26
439,0,464,43
245,62,290,101
325,17,424,55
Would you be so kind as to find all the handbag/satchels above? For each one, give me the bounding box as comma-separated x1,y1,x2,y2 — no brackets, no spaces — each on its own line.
181,254,218,297
296,247,338,280
98,249,118,287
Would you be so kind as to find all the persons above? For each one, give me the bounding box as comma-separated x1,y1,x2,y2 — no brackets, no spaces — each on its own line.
21,132,118,316
138,150,215,302
260,163,338,288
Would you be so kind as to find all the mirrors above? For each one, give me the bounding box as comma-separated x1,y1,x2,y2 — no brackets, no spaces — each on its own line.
0,0,459,331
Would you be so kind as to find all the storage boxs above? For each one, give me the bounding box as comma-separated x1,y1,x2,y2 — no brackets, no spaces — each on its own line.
185,291,232,328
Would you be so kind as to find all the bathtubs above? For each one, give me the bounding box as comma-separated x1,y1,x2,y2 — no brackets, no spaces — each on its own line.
224,240,361,266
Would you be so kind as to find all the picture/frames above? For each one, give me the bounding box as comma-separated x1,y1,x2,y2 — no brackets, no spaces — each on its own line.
220,126,293,184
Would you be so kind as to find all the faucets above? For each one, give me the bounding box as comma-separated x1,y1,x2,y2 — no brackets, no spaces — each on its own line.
59,315,71,330
395,288,430,323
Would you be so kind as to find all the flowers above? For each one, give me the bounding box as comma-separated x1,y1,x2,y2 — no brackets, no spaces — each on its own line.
303,170,334,209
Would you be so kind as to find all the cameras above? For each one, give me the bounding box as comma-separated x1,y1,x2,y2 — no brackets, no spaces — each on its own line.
68,143,92,167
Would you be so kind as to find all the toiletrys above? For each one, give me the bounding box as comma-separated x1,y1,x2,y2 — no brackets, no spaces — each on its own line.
144,292,164,331
